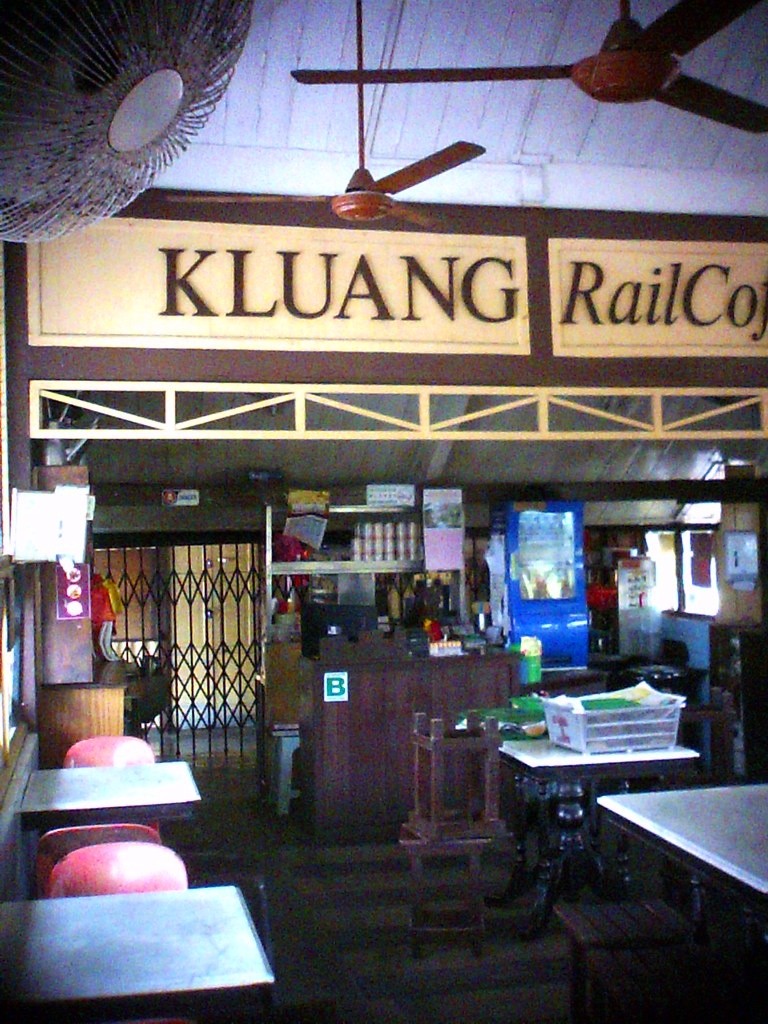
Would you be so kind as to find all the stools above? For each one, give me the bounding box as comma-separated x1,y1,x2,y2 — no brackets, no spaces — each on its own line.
553,899,696,1024
267,737,299,816
399,827,493,957
402,711,513,845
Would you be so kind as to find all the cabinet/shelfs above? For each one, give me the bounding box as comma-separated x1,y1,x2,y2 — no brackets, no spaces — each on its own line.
262,506,466,778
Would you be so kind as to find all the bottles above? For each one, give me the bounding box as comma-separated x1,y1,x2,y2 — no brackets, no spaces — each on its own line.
402,584,415,619
387,584,401,618
287,587,301,637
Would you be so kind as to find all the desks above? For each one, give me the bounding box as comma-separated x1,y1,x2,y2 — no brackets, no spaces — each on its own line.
0,885,275,1024
456,715,699,942
597,784,768,1024
20,761,202,831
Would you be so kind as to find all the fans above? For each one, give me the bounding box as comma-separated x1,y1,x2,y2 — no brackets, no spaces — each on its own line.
165,0,486,233
291,0,768,136
0,0,253,243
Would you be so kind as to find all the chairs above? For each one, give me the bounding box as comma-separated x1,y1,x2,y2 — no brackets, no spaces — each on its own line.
36,824,162,899
64,736,160,832
44,842,189,900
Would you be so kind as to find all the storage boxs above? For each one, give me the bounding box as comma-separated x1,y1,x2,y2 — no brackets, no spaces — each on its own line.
542,699,686,755
